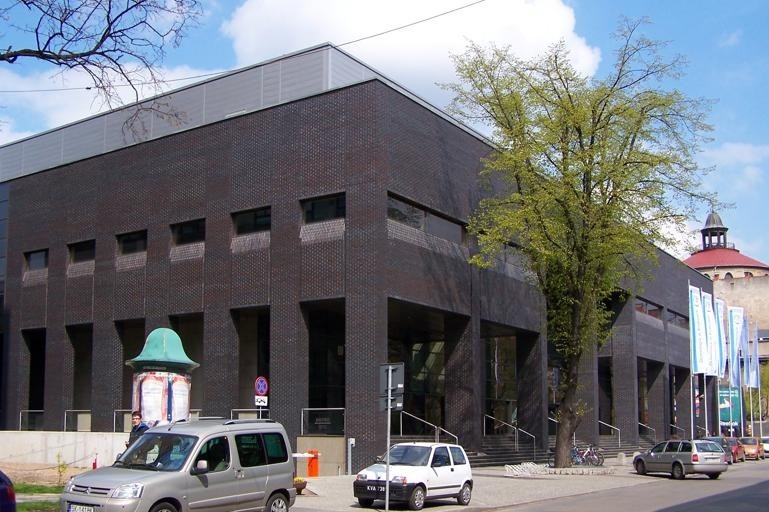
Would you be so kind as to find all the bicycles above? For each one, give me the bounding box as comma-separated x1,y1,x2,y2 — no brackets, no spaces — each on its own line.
546,442,608,469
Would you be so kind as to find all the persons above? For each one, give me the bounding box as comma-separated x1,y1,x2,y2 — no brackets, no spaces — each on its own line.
124,412,156,467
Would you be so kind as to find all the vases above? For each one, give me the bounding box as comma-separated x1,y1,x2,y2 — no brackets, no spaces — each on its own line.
295,481,307,496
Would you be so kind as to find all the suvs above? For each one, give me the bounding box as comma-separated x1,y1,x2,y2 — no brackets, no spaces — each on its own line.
61,414,299,512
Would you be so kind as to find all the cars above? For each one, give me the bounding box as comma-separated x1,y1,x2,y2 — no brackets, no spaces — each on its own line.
0,470,17,511
709,434,769,464
632,437,730,483
349,440,476,508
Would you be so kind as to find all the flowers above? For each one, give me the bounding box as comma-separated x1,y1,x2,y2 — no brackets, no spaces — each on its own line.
292,474,307,485
